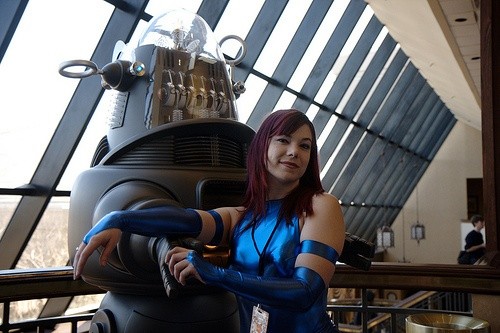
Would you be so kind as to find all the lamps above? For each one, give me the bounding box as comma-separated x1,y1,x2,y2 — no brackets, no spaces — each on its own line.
377,149,394,252
410,155,425,243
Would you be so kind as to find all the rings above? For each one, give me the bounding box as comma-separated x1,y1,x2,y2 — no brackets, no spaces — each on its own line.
75,246,79,251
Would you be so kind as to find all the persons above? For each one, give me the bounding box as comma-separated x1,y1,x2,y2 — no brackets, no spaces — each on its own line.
355,287,390,333
464,214,486,266
71,109,345,333
